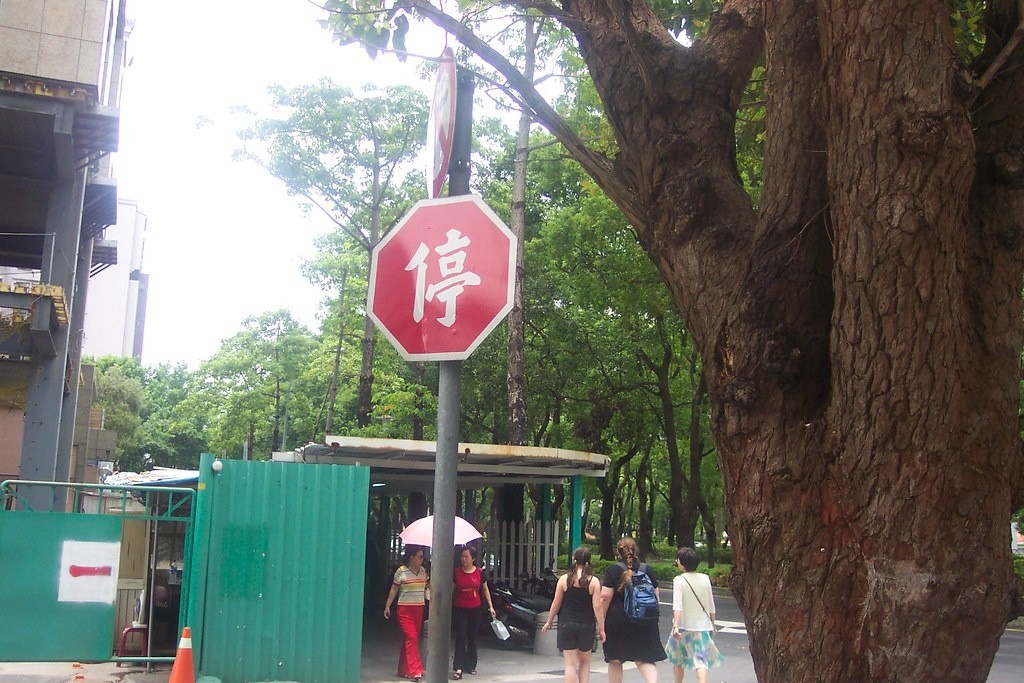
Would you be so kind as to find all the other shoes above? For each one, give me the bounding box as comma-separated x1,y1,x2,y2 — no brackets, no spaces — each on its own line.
414,676,422,683
453,672,462,679
469,671,477,675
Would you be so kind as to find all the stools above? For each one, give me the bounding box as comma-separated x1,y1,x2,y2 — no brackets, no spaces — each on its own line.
117,624,148,667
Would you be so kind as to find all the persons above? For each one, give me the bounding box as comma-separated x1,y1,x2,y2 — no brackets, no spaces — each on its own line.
601,538,667,683
541,547,606,683
452,546,496,680
664,548,724,683
147,577,169,620
383,545,430,681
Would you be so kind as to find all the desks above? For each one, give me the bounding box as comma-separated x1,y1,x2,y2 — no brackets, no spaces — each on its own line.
168,579,181,606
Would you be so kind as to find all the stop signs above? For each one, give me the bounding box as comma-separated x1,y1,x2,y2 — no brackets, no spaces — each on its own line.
365,193,518,360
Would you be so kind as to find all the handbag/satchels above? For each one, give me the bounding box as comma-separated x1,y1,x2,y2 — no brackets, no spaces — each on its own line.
491,615,510,640
424,588,430,600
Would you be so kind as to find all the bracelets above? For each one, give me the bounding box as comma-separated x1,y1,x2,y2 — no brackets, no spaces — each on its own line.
386,606,390,607
673,627,677,628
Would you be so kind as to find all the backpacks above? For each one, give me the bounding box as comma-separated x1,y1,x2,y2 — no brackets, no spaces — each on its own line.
616,562,659,621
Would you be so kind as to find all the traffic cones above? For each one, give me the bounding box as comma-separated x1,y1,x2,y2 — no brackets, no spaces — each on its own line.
166,627,196,683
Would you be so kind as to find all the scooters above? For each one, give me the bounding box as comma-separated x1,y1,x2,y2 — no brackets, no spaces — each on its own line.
484,557,560,649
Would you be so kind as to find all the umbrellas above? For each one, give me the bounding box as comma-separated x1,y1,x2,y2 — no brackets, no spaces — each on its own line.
399,515,483,547
